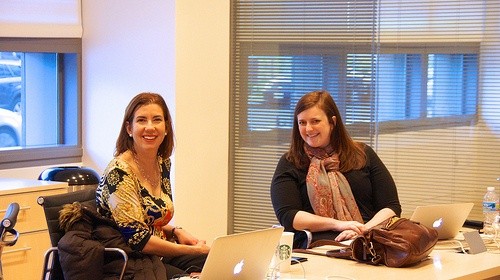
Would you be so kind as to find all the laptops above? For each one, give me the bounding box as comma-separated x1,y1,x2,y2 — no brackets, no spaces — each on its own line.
199,226,284,280
411,203,474,240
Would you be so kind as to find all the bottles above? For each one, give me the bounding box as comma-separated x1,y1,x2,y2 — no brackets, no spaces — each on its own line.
483,187,500,243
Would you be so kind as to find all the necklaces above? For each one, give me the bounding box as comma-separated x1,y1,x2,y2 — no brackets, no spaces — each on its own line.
133,153,160,185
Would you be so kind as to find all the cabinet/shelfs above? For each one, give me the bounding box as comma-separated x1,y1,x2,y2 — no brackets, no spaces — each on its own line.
0,180,79,280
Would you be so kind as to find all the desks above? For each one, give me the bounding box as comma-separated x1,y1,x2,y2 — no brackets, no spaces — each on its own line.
181,221,500,280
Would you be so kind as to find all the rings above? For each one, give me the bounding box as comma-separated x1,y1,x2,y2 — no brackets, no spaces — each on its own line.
355,233,357,235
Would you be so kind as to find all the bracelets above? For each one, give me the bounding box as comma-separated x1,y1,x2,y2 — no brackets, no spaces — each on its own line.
172,227,182,234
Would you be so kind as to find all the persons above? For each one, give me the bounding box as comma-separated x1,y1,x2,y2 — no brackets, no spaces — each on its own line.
96,93,210,280
270,91,402,250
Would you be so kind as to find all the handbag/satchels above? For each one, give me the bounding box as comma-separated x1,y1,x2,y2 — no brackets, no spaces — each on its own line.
349,215,439,268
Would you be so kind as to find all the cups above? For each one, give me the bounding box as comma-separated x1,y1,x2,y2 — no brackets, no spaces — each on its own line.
272,232,295,272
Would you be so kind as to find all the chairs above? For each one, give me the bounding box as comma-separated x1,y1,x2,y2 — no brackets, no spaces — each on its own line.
0,188,125,279
